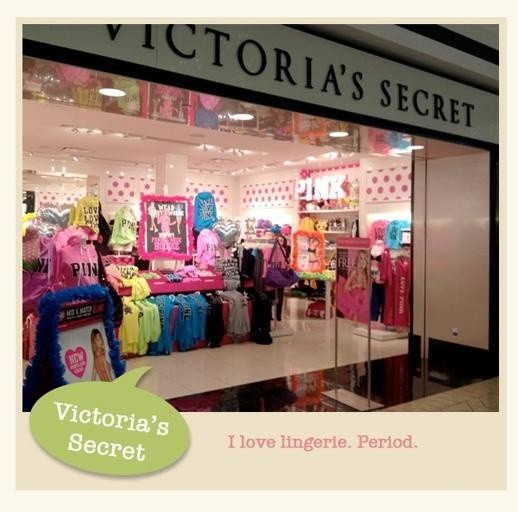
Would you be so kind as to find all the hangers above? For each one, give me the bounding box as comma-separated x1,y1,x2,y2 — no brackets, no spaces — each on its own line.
130,297,144,312
118,266,140,282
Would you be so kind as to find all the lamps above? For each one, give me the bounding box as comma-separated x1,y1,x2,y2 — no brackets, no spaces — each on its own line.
99,88,126,97
408,146,423,150
231,113,255,122
329,131,348,139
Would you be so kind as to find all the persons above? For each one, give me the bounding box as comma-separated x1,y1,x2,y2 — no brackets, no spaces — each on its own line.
336,250,369,324
308,237,319,269
264,235,299,321
91,328,113,383
148,201,183,235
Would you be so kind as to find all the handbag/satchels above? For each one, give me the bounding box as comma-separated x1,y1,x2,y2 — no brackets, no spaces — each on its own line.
305,299,334,319
264,238,300,289
